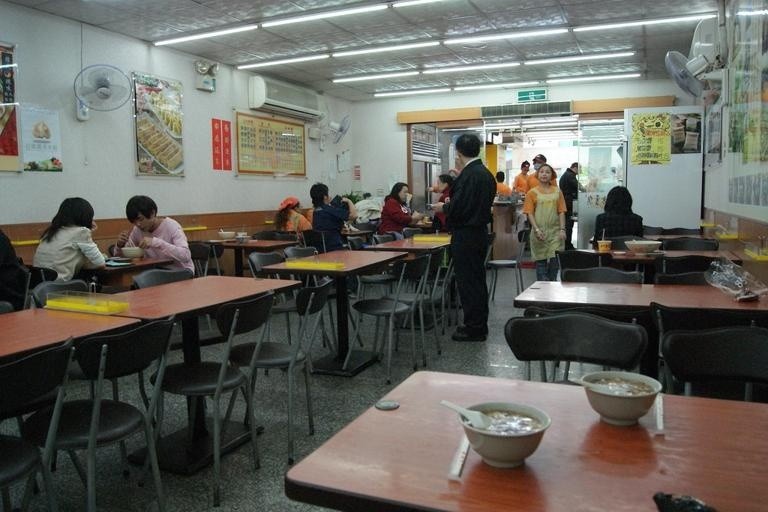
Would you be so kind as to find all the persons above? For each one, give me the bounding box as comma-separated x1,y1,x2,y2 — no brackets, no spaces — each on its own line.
33,197,106,283
113,196,195,290
0,230,29,313
494,153,585,283
426,134,497,341
277,181,425,252
594,186,643,250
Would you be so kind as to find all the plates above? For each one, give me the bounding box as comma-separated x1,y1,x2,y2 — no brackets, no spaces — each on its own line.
136,92,183,175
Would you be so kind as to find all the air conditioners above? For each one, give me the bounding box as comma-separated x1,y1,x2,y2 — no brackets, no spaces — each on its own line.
248,76,326,121
689,18,728,69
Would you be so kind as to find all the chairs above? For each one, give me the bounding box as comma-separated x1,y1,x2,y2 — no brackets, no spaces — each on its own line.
222,279,337,435
2,336,74,511
663,325,768,404
512,221,767,328
504,314,646,387
486,240,528,302
162,294,274,501
342,254,429,385
0,221,501,338
33,320,172,512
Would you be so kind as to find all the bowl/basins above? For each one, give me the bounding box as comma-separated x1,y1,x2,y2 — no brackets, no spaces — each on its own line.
458,401,553,469
121,246,145,260
218,232,251,244
624,240,663,255
581,371,663,426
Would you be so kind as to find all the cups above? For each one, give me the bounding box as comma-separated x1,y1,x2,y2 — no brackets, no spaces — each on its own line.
597,241,612,253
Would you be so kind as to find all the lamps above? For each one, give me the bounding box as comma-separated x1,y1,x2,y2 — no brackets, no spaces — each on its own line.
372,80,540,100
194,59,219,91
150,0,437,48
331,50,641,81
236,14,716,70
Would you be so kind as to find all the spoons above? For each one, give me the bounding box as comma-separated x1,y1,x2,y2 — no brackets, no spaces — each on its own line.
442,399,492,429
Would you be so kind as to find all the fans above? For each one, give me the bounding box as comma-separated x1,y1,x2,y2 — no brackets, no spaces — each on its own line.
319,114,352,151
665,50,729,106
73,64,132,121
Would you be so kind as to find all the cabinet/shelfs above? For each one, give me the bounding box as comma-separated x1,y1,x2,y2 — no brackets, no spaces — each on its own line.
413,155,443,213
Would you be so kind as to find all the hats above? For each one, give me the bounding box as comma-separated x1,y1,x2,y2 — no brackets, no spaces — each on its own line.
533,154,546,163
522,160,530,167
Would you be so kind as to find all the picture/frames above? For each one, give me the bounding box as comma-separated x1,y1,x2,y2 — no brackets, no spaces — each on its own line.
236,110,306,176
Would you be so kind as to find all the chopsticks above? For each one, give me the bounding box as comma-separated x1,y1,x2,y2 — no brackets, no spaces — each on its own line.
450,433,470,478
128,236,137,248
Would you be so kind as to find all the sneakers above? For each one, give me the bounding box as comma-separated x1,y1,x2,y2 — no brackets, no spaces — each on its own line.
452,326,488,341
